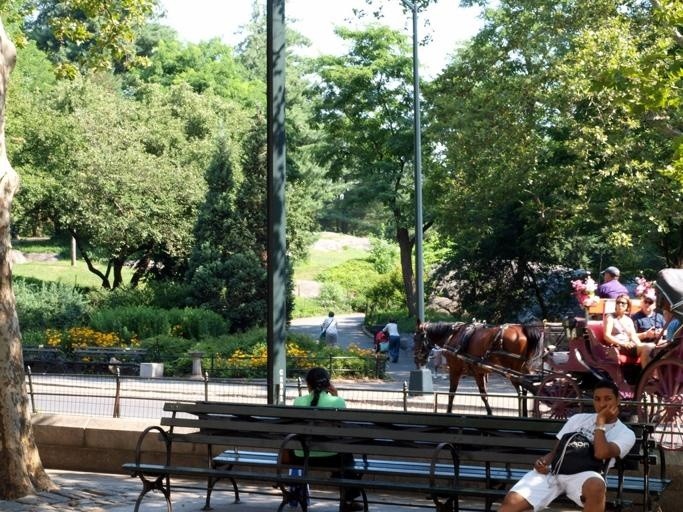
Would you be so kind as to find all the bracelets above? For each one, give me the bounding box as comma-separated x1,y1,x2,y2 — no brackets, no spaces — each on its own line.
593,426,606,433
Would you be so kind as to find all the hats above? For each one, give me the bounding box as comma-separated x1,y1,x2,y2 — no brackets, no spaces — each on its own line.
641,293,656,302
600,265,620,278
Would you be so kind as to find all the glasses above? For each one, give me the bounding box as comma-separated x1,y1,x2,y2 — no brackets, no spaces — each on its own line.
616,301,627,304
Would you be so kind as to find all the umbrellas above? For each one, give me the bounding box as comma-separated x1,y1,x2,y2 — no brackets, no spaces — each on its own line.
654,268,683,344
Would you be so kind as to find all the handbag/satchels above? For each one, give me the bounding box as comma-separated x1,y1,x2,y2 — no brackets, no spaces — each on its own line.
549,430,604,475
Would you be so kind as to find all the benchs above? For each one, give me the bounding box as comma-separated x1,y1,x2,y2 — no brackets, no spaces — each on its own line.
581,297,640,367
119,398,672,512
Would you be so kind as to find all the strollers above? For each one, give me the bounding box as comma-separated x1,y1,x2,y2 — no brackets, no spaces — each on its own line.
372,331,393,365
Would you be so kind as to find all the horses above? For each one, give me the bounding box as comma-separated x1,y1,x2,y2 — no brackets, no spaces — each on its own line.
413,317,544,417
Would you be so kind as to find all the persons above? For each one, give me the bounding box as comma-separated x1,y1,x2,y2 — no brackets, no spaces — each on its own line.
498,382,636,512
322,312,338,346
292,367,363,512
598,266,681,384
380,317,400,362
429,344,442,379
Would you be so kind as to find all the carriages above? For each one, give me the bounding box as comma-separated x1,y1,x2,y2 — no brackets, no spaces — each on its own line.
413,269,683,452
